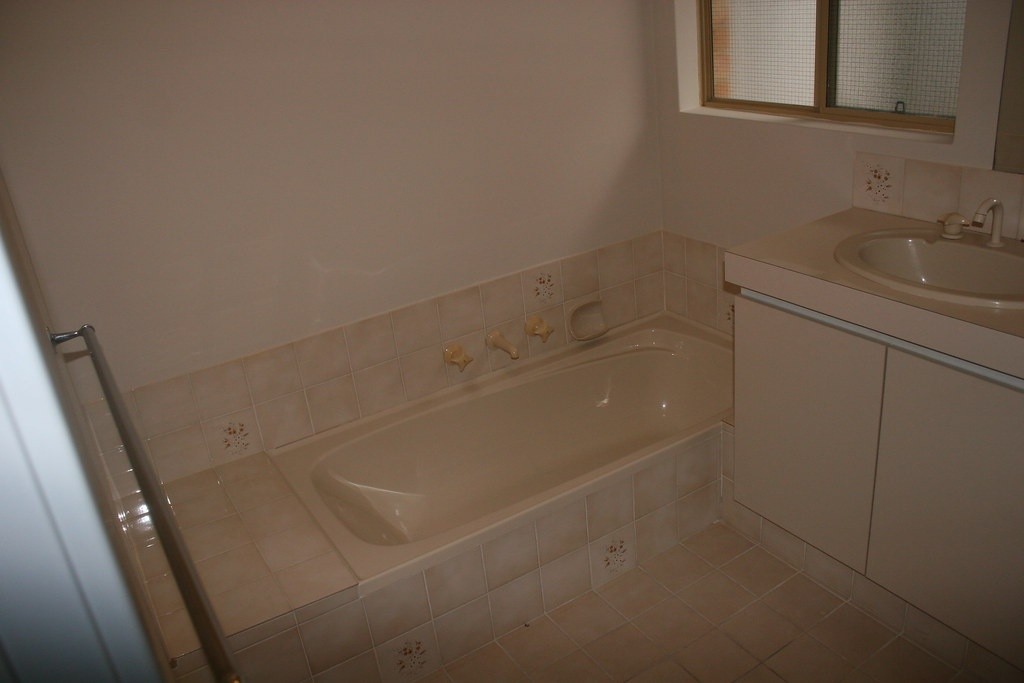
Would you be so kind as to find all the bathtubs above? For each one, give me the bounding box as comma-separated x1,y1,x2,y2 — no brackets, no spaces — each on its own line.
267,310,734,632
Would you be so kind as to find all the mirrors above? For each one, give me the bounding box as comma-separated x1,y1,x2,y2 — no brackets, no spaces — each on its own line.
992,0,1024,175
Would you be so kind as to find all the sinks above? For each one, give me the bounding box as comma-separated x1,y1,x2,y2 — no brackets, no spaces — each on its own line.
832,227,1024,311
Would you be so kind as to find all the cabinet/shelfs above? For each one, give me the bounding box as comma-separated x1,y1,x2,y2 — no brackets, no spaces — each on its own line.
732,286,1024,673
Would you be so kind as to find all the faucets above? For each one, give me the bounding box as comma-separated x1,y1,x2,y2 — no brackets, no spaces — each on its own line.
486,329,521,360
970,196,1004,248
